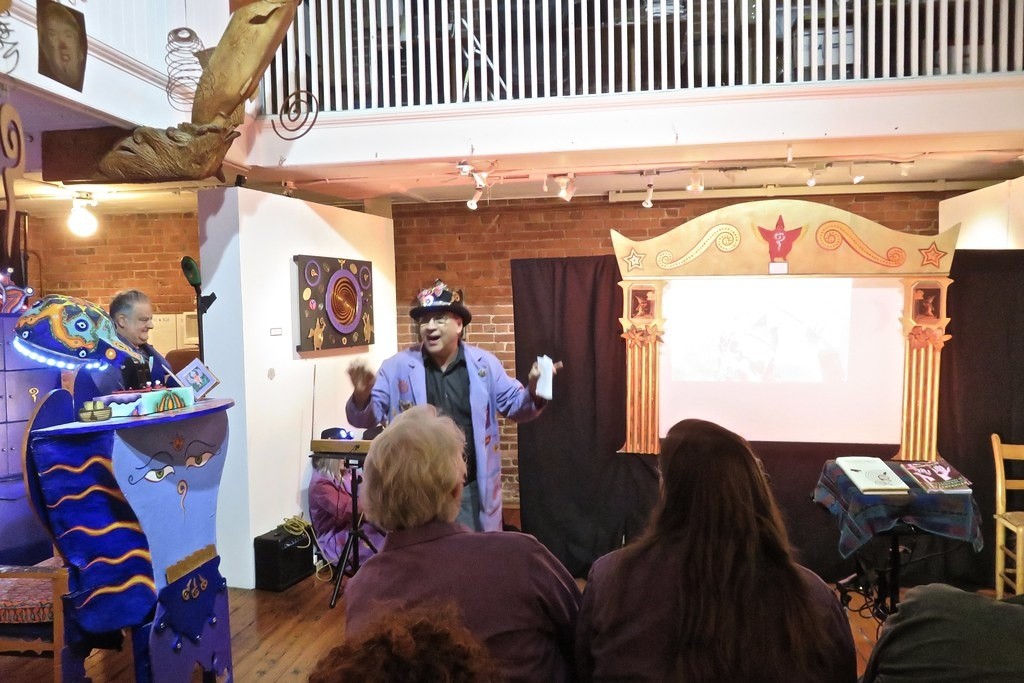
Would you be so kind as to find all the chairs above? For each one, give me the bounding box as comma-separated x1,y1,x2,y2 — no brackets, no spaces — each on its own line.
991,433,1024,600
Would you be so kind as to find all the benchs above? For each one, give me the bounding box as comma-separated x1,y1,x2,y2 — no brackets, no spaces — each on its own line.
0,555,67,683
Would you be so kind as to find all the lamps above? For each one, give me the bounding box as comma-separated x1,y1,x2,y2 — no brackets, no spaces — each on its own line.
640,170,661,208
807,168,816,187
687,166,704,191
554,172,577,202
849,161,864,184
457,160,485,210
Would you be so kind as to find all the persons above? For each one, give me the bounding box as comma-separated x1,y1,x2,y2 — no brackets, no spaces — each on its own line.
344,402,583,683
73,290,180,420
37,3,84,91
574,420,858,682
859,582,1024,682
308,428,387,572
346,278,563,532
308,599,506,683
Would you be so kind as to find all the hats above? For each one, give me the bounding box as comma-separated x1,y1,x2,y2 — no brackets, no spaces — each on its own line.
321,428,347,440
410,277,472,327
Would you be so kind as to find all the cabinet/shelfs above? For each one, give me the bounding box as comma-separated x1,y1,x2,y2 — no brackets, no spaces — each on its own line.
0,313,61,563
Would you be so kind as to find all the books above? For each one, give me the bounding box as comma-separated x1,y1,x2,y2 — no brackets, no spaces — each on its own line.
836,456,910,495
900,458,973,495
